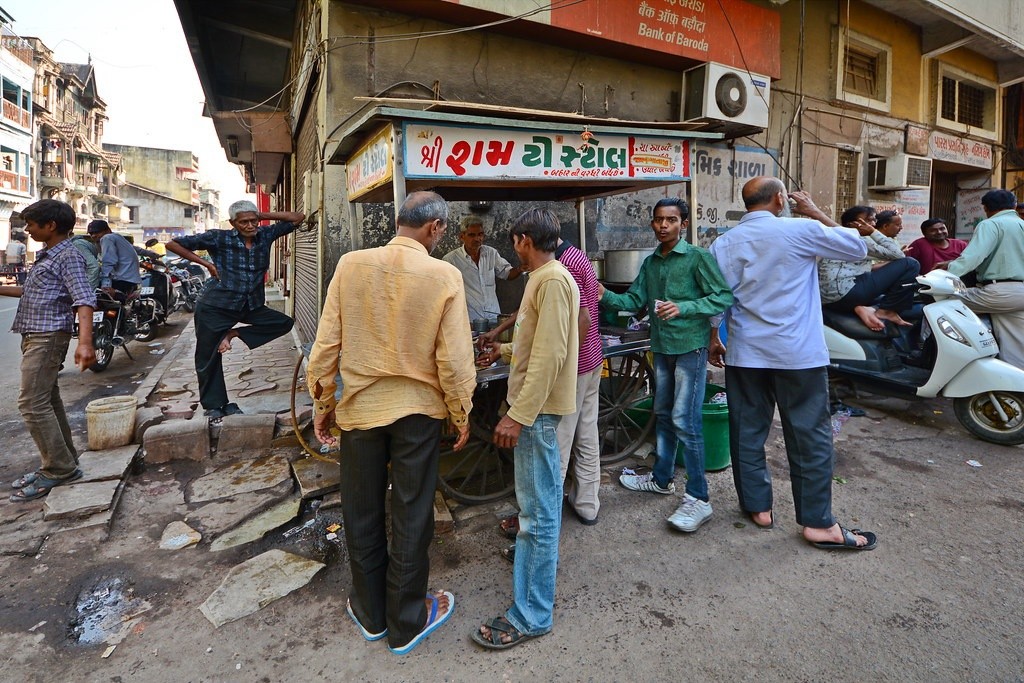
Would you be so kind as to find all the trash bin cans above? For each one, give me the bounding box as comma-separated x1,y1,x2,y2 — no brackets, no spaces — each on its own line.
678,383,730,472
85,396,138,450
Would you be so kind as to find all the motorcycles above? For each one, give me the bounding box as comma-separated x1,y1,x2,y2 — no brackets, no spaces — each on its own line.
72,237,210,372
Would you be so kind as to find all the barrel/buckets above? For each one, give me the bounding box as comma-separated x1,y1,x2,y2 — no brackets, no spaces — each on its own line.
597,376,730,472
84,395,139,450
496,314,514,342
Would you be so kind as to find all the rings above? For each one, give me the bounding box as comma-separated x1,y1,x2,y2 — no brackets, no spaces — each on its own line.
486,358,490,362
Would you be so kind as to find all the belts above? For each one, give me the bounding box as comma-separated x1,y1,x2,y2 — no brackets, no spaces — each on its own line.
981,279,1023,286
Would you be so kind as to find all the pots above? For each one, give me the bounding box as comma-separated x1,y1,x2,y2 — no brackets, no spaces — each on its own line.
588,257,604,280
600,247,657,283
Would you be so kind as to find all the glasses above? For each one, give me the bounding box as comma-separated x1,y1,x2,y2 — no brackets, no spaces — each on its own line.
893,210,899,215
866,217,877,223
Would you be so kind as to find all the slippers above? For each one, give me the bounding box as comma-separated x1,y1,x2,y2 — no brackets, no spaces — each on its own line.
388,590,455,654
11,459,79,489
563,495,598,525
751,508,774,528
810,522,877,550
203,407,225,424
222,403,243,416
10,469,83,502
498,514,519,540
346,598,388,641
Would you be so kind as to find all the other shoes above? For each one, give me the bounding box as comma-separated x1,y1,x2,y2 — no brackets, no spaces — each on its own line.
830,402,866,417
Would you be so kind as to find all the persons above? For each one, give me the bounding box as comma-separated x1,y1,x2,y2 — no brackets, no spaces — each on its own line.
818,207,920,331
871,210,912,292
442,218,521,322
5,236,27,280
0,199,96,503
307,191,476,656
70,220,159,338
947,190,1024,372
472,208,580,650
705,176,876,550
499,219,600,538
905,218,975,305
165,201,305,425
598,199,733,533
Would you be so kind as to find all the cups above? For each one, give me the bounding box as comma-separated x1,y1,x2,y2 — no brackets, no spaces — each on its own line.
787,197,798,209
473,318,490,332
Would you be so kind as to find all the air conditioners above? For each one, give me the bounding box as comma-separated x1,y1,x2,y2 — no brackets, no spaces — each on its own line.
867,155,932,191
680,61,770,133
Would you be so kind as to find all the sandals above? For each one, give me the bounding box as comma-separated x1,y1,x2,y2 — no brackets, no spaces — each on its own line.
501,544,516,562
472,617,552,650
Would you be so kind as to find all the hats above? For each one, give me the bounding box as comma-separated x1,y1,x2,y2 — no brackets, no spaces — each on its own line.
87,220,109,234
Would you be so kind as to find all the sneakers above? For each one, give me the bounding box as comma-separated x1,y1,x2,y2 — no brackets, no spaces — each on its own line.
667,493,713,532
619,471,675,495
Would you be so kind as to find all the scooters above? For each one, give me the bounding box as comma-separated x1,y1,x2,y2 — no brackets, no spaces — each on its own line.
823,267,1024,444
